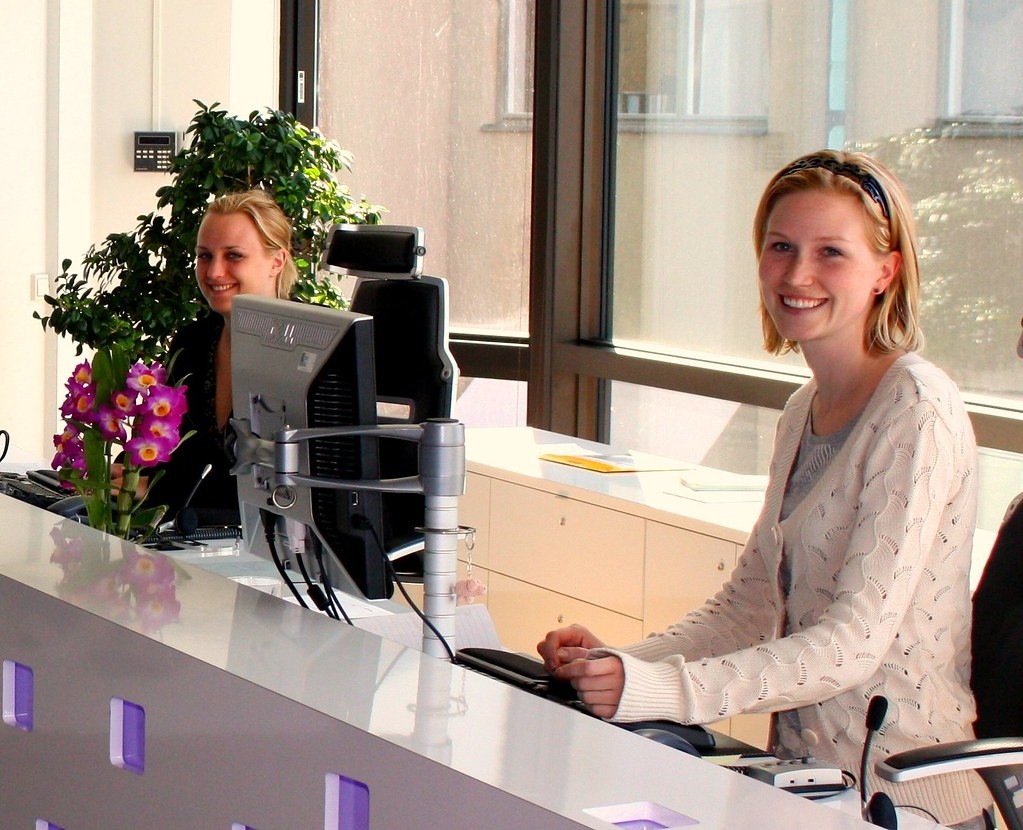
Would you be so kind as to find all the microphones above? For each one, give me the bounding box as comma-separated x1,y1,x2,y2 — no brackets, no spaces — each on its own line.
184,464,212,507
859,696,890,804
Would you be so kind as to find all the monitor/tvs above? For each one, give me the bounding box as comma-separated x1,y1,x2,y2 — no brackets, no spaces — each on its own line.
231,294,397,604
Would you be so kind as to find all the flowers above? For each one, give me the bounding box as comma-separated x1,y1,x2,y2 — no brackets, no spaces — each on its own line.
47,520,192,633
51,346,197,545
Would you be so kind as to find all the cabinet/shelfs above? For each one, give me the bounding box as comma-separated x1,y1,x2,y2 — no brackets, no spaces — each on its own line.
392,415,773,754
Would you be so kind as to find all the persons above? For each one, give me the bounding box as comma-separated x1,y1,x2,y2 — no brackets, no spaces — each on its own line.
536,149,995,830
107,187,300,506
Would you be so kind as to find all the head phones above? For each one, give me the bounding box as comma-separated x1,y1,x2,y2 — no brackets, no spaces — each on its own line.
155,508,203,550
862,792,898,830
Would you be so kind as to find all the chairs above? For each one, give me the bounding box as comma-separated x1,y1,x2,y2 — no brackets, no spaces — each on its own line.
875,497,1022,830
317,223,461,583
